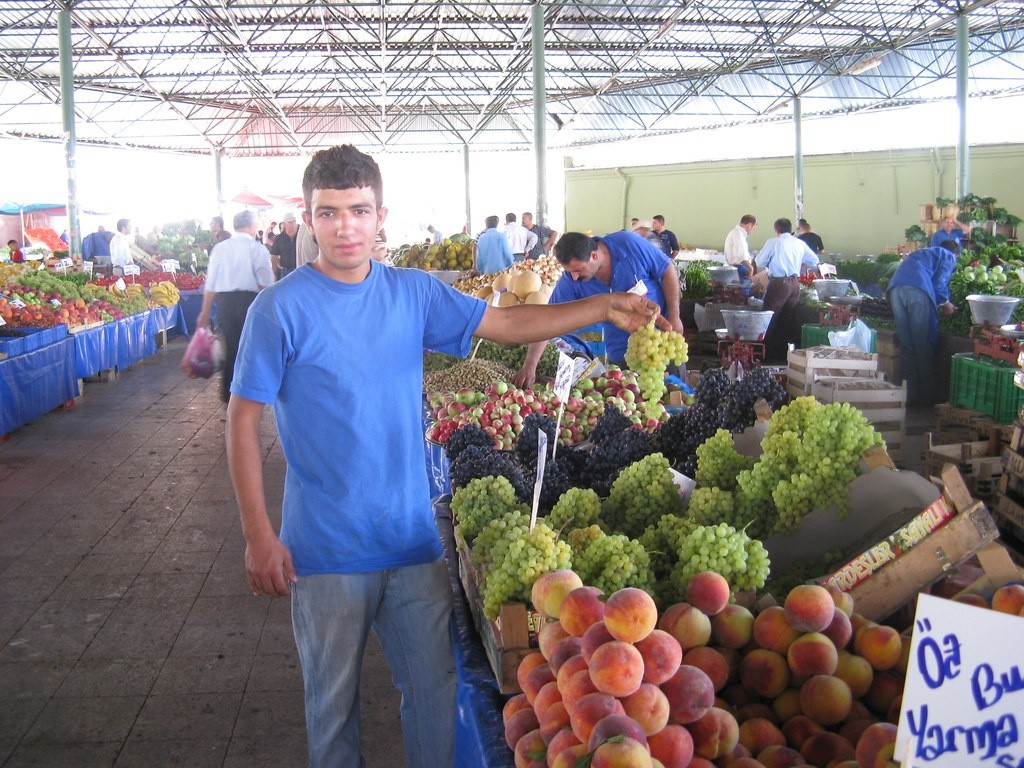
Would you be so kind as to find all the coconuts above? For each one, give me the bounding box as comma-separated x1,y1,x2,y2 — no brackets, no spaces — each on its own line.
390,238,472,270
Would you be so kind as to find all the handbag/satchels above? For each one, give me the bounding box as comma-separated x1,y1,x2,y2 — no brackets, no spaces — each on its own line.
181,327,217,379
826,317,871,353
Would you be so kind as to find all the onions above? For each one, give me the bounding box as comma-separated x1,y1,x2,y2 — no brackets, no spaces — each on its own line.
452,254,566,297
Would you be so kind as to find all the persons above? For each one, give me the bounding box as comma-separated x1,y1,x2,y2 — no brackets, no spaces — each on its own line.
196,210,317,423
60,229,69,245
110,217,136,268
7,239,23,262
427,226,443,245
752,218,819,362
522,213,558,259
931,214,971,254
424,238,432,244
225,140,673,768
723,214,758,299
504,213,539,264
97,225,105,231
472,216,514,277
886,241,960,402
790,219,825,256
630,214,678,260
512,228,690,389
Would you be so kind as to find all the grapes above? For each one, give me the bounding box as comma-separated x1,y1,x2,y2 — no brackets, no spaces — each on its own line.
624,321,689,418
14,270,147,315
445,363,887,605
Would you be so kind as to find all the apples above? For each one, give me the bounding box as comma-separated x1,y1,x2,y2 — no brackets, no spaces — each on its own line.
426,365,670,449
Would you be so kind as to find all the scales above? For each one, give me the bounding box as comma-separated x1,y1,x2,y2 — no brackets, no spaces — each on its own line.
812,278,866,326
715,309,775,380
707,267,754,306
965,294,1024,367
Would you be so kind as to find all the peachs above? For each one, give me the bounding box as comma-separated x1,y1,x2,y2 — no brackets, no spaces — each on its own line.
0,284,124,328
955,585,1024,617
504,568,912,768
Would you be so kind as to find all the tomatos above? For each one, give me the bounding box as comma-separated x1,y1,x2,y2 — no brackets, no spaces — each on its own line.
800,272,823,286
95,269,205,289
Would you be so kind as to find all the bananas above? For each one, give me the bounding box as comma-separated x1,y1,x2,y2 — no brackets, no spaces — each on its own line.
109,280,181,309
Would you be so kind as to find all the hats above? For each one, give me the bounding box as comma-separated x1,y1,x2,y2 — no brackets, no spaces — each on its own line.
283,213,296,222
633,221,652,230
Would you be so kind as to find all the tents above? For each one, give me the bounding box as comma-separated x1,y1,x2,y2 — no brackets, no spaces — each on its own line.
0,197,115,259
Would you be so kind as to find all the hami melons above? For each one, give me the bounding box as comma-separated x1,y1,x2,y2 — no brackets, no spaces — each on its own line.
474,271,552,307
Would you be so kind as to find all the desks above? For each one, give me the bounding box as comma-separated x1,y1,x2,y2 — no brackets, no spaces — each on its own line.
0,286,221,437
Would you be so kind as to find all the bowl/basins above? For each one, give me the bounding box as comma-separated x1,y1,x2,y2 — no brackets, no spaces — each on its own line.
714,329,728,339
707,266,739,285
812,278,852,301
720,309,775,342
964,294,1019,326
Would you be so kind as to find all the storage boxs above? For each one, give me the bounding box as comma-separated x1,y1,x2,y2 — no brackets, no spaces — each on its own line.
949,352,1024,424
63,378,84,409
155,331,167,348
451,400,1011,697
86,366,120,383
886,204,1019,258
801,324,877,353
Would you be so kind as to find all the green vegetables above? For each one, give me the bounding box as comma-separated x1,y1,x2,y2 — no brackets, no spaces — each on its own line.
423,337,559,377
836,195,1024,337
683,259,724,297
157,227,213,266
